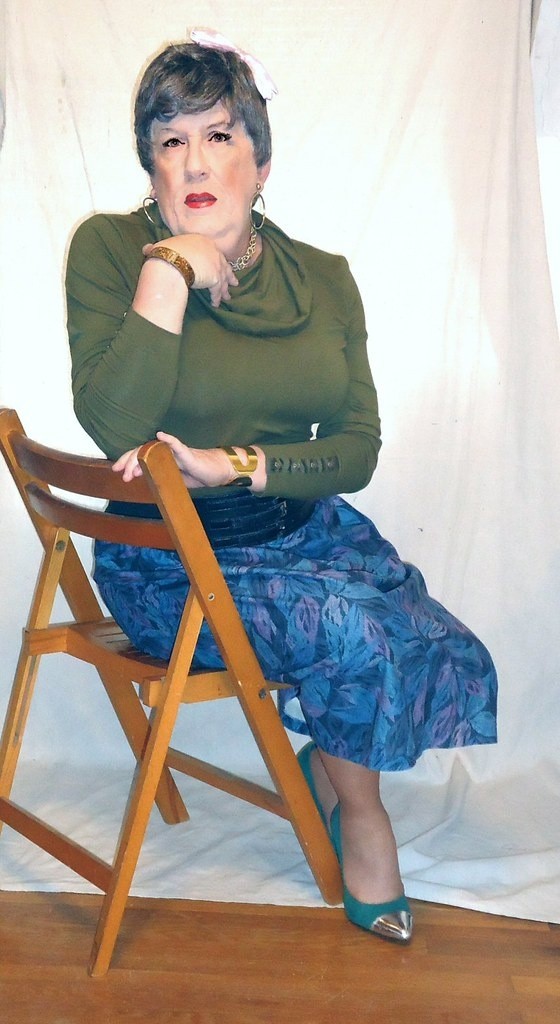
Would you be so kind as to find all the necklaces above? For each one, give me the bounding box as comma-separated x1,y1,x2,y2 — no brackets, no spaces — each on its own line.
227,218,257,271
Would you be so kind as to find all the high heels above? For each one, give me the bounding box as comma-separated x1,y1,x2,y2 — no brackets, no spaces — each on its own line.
295,741,336,851
330,802,413,941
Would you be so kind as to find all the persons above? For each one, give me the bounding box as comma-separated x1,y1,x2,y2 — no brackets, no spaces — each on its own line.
64,41,499,943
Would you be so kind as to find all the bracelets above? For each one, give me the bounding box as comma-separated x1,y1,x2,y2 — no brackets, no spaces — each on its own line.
143,246,195,289
216,445,258,486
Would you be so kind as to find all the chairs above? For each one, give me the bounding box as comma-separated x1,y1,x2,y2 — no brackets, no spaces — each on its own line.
0,407,345,978
